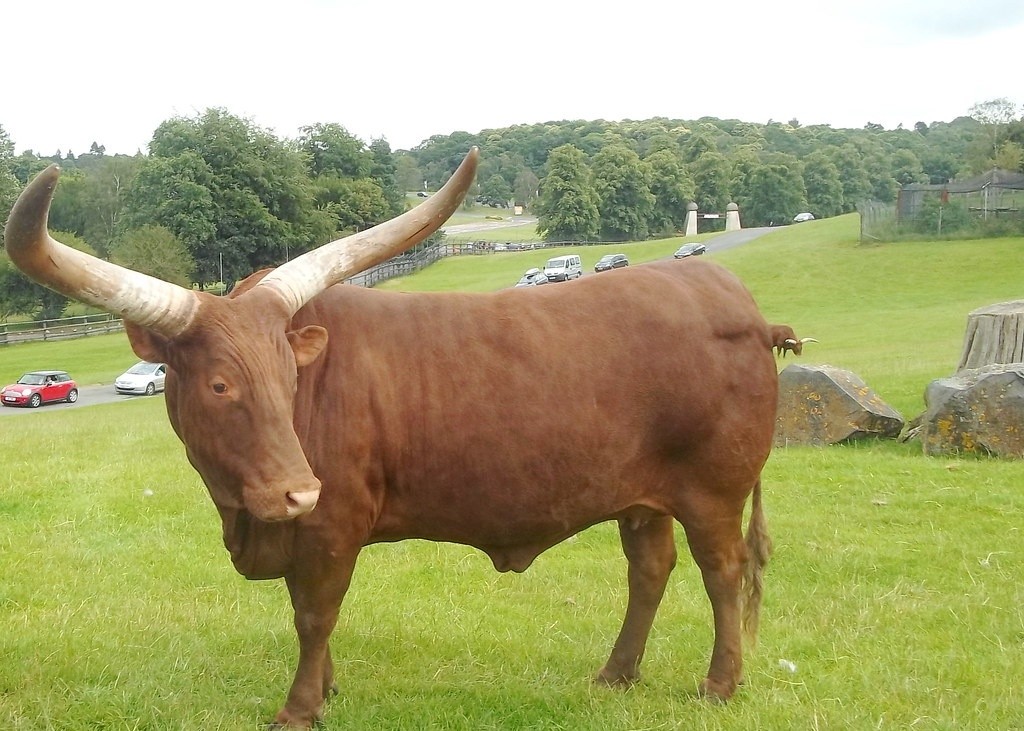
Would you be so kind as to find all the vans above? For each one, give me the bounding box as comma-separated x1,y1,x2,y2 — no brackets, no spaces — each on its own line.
543,254,583,282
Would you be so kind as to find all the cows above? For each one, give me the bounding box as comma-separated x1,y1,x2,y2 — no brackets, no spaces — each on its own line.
2,144,819,731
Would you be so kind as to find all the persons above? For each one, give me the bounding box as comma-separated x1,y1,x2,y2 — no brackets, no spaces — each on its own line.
46,375,57,385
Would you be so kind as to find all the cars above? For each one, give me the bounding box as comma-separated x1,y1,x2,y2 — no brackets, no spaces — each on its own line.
594,253,629,273
793,212,816,222
515,267,547,289
674,242,706,260
114,360,166,396
0,371,79,408
